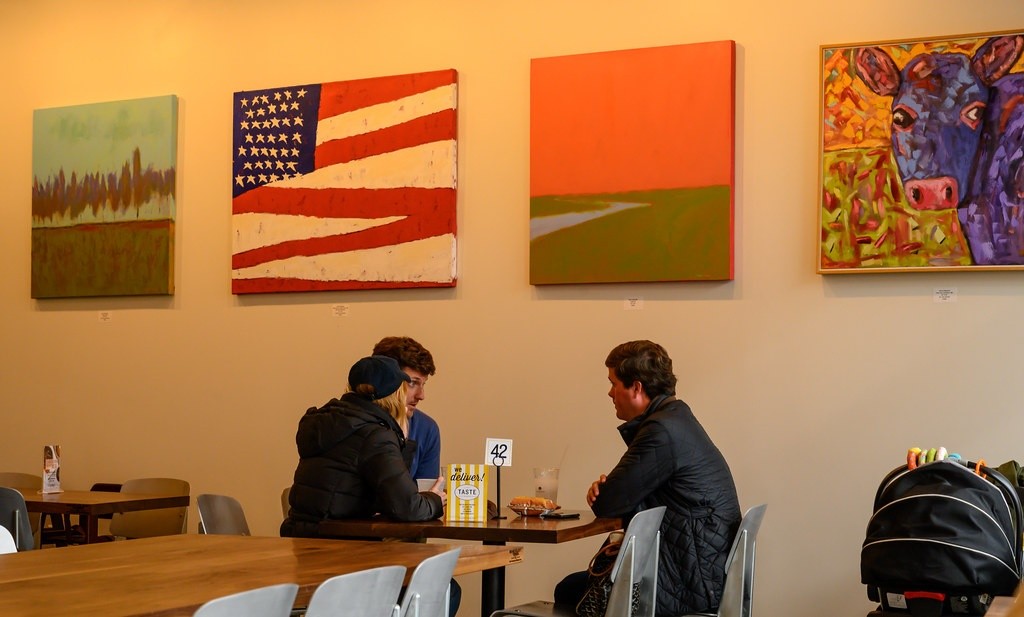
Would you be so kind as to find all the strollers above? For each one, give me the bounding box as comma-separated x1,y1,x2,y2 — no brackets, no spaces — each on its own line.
860,446,1024,617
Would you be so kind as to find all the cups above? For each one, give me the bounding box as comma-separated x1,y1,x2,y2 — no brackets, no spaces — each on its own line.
532,467,559,504
415,478,444,495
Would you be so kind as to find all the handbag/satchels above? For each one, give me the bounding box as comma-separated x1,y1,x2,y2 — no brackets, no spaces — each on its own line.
575,532,640,617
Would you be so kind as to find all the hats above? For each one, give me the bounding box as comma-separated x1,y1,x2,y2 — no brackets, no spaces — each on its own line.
348,355,411,401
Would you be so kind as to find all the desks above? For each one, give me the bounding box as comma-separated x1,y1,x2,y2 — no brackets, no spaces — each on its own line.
25,490,191,545
0,534,524,617
319,508,624,617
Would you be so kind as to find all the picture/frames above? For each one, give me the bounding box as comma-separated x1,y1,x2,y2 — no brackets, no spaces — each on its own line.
816,29,1024,274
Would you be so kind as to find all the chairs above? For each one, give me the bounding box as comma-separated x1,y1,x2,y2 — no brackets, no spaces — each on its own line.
303,566,407,617
398,546,461,617
196,493,251,536
682,503,768,617
0,472,190,555
192,583,299,617
489,506,667,617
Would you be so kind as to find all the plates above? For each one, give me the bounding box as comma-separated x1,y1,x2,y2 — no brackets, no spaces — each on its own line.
507,503,561,516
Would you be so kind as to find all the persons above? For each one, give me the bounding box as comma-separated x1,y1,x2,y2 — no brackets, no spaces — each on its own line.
554,339,743,617
372,337,441,479
280,356,462,617
